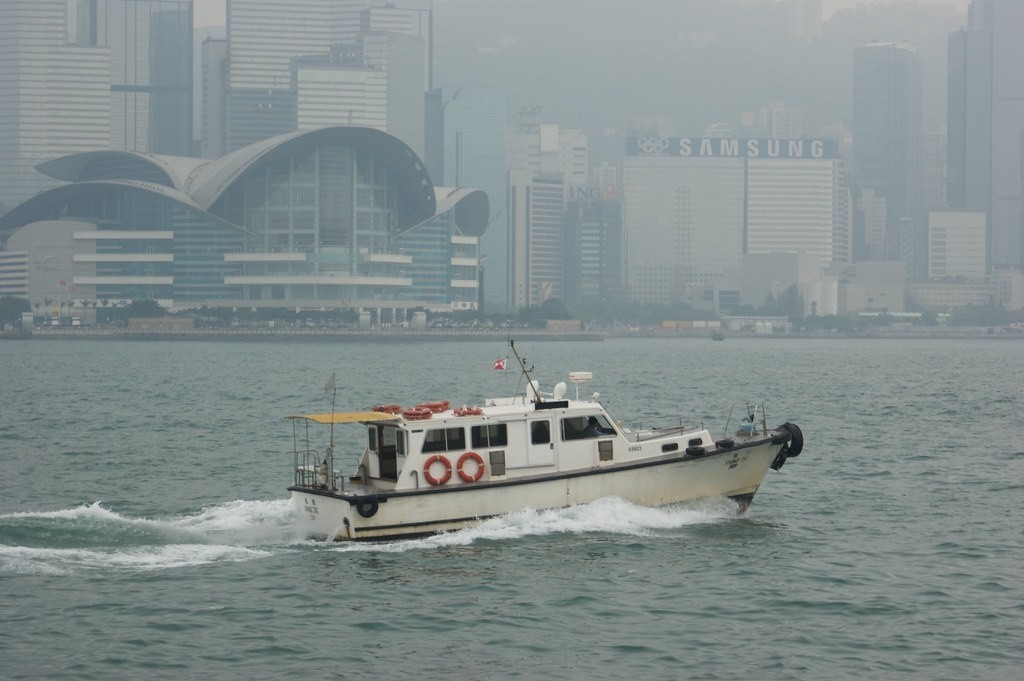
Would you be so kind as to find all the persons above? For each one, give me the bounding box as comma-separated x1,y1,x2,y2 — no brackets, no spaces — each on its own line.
580,417,609,439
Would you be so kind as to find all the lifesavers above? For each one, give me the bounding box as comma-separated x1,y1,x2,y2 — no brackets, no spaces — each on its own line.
356,496,378,518
423,455,452,486
454,407,482,416
373,401,452,421
777,422,804,458
715,440,734,448
770,448,789,471
457,452,485,482
686,446,705,455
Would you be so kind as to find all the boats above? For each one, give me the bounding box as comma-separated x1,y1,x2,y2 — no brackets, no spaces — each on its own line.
283,335,803,543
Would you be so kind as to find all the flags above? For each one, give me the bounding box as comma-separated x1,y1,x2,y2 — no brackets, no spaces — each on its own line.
494,360,506,370
